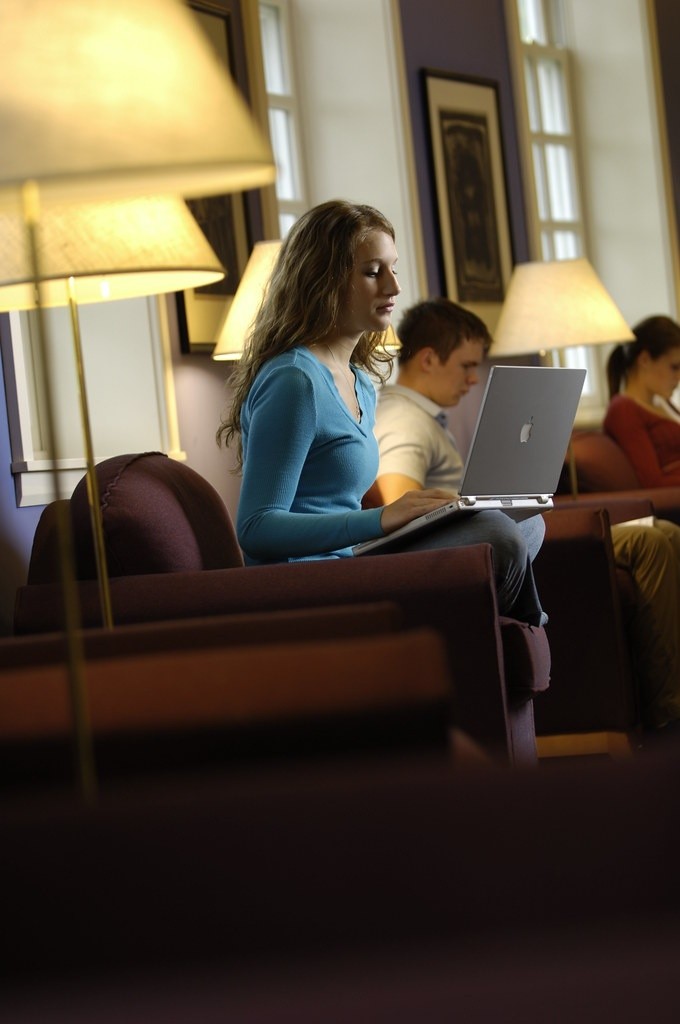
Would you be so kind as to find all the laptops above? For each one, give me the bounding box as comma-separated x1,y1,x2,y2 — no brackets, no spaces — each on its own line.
352,365,587,557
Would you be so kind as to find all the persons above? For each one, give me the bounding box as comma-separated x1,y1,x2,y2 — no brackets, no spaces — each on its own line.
218,199,549,630
373,298,680,720
602,314,680,525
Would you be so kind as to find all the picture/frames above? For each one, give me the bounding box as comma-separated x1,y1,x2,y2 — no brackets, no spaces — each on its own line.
171,1,254,356
416,67,517,303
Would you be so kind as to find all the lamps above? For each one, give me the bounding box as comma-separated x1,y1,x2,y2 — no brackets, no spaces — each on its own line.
0,0,278,797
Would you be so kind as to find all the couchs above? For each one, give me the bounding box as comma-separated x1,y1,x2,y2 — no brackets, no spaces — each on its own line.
7,428,680,778
